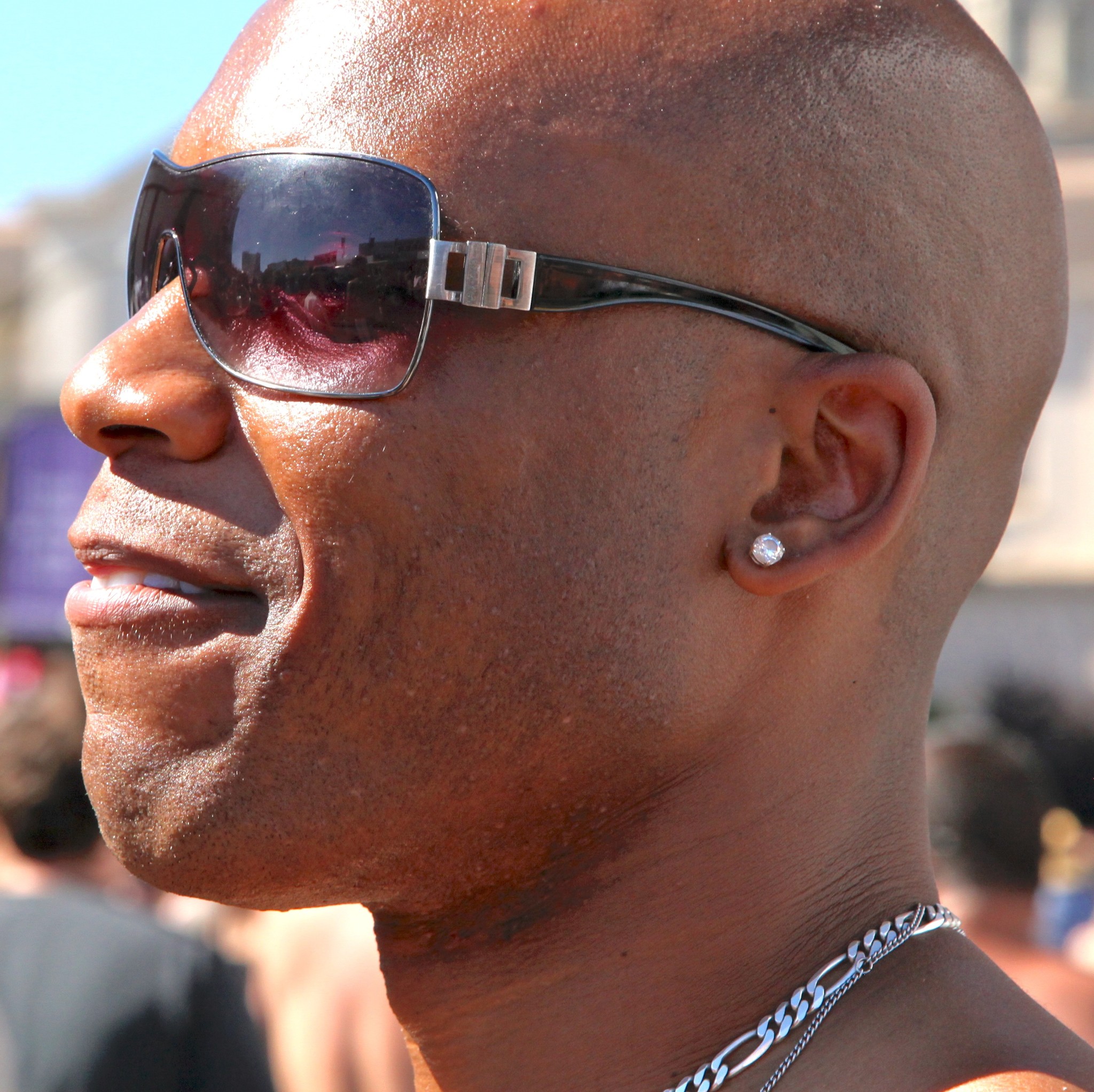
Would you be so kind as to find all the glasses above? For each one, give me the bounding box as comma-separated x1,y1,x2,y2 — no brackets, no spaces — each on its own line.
122,149,858,401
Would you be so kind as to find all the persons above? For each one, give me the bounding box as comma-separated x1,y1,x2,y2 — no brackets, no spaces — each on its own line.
59,0,1094,1092
0,640,1094,1092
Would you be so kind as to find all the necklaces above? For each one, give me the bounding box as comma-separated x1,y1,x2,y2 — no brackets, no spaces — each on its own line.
660,901,966,1092
